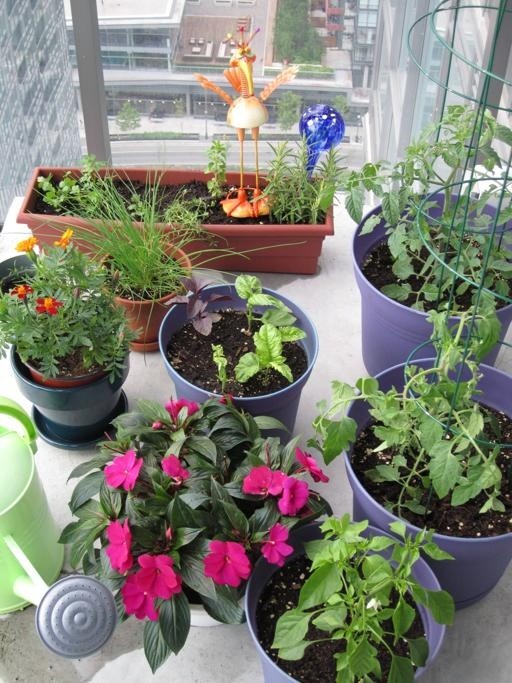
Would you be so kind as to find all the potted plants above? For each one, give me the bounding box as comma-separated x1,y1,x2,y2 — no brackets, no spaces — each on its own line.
1,103,512,683
